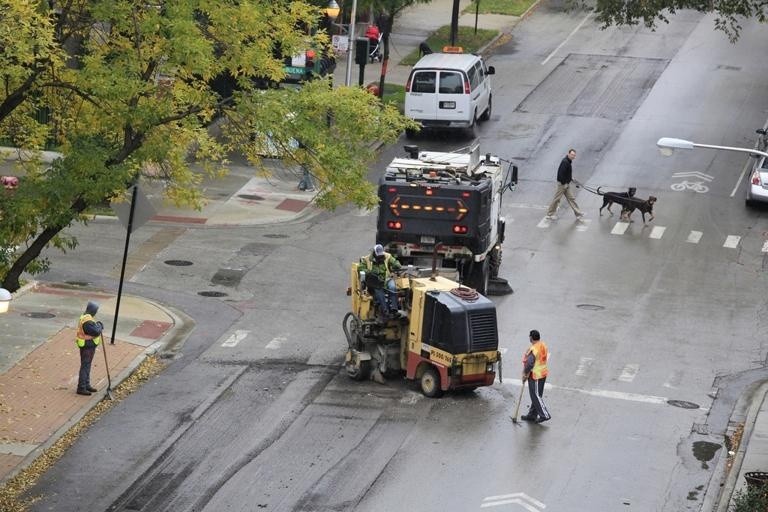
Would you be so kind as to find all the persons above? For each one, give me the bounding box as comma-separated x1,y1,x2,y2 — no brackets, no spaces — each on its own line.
357,244,401,319
521,330,551,423
546,149,584,220
375,8,390,59
75,301,103,395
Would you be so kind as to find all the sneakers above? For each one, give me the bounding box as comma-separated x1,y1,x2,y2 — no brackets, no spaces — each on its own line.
389,309,401,318
546,216,559,221
77,388,92,395
521,413,537,420
577,212,587,219
87,385,97,392
535,417,545,424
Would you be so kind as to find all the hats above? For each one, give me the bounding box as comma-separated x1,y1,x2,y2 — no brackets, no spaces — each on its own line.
373,244,385,256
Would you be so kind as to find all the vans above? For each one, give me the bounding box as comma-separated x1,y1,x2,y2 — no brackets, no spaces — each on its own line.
401,44,496,141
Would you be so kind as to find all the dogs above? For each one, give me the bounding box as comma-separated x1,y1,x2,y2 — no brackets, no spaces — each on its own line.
597,185,636,218
623,195,658,224
419,42,432,57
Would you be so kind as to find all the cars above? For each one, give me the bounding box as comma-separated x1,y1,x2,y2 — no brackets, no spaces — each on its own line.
755,120,767,152
743,143,767,202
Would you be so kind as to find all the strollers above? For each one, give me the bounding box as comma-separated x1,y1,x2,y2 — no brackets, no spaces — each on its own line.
362,25,383,62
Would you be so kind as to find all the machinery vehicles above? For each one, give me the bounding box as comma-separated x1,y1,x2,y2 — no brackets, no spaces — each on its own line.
343,247,501,393
373,136,519,297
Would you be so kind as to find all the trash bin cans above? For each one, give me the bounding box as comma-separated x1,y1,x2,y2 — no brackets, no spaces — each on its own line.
744,472,768,492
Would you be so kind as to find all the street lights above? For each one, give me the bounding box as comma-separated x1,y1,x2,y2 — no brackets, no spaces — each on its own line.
325,0,340,128
656,137,767,160
304,46,319,81
